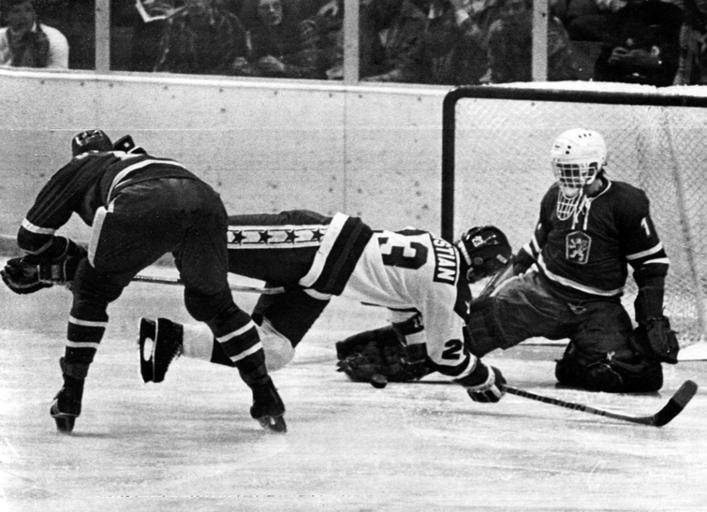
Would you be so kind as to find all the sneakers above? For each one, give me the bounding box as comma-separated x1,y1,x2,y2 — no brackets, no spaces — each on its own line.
137,317,182,384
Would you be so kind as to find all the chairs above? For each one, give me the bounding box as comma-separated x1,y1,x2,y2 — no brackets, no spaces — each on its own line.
0,0,707,86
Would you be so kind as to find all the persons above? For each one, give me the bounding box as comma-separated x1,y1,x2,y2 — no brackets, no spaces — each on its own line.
339,125,680,395
94,207,512,403
1,1,706,86
0,128,286,418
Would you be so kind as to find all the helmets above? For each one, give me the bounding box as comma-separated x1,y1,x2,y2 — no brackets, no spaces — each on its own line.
72,130,112,158
462,225,512,283
551,127,607,221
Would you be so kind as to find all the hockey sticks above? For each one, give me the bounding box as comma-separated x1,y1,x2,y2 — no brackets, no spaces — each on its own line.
498,381,697,426
132,275,305,294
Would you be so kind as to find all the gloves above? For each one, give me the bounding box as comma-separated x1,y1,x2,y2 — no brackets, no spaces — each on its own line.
467,366,507,403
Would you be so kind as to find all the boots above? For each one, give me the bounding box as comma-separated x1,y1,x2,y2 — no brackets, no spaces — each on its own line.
50,357,93,415
240,363,285,417
